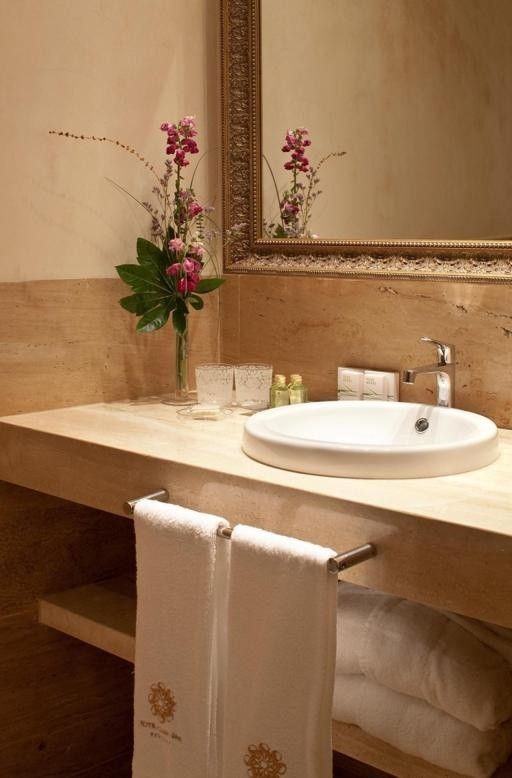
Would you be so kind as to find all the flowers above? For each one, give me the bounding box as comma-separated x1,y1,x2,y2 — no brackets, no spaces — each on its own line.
47,115,247,393
261,124,347,238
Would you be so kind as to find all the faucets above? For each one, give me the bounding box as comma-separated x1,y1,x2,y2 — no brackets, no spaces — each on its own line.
402,336,457,407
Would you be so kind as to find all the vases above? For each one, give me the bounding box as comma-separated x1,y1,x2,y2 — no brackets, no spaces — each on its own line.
159,301,197,404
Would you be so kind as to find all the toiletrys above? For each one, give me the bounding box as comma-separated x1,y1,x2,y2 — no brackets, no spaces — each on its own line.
271,374,308,408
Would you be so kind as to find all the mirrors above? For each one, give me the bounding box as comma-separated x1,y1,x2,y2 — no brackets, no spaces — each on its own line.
218,0,510,285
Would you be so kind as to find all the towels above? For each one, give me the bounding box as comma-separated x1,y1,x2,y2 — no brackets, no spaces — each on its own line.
334,675,508,778
219,523,339,778
130,499,234,778
332,582,507,733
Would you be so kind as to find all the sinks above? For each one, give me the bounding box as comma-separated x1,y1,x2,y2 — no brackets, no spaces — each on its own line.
243,398,499,476
